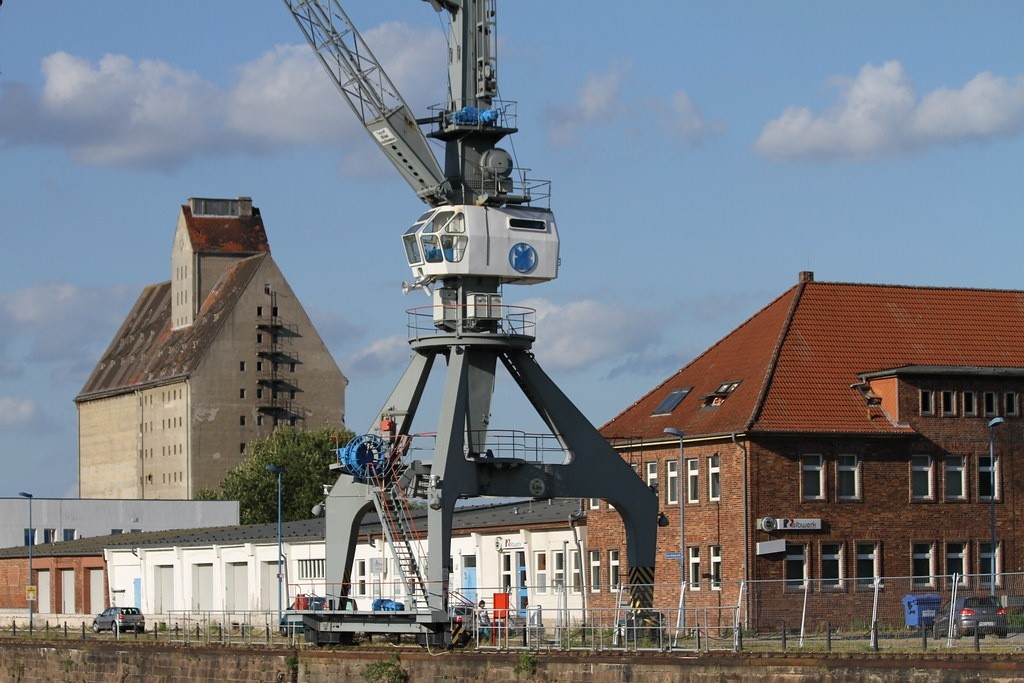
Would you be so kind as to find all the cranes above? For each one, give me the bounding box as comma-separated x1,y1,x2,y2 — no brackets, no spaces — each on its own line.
281,0,658,648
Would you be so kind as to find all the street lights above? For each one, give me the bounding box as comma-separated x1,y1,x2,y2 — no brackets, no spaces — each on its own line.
663,428,687,639
988,418,1005,596
19,492,33,622
264,465,282,624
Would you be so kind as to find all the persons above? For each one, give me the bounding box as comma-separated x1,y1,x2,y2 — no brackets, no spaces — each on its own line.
475,600,492,642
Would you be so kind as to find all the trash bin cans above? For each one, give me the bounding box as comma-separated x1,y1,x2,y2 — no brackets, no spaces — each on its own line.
372,598,404,612
902,593,942,630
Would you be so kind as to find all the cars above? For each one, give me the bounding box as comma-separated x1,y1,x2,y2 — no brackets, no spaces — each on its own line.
933,596,1008,640
93,607,145,634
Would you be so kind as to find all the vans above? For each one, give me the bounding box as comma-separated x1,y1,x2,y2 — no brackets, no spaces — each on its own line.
448,605,477,634
280,598,358,637
